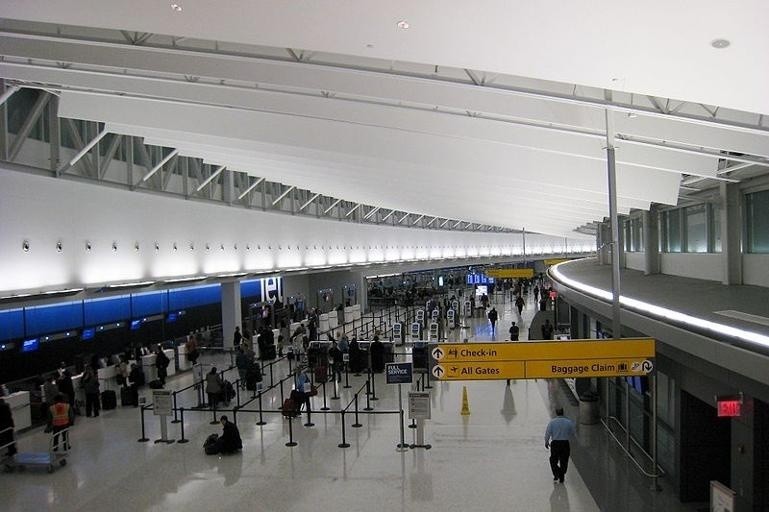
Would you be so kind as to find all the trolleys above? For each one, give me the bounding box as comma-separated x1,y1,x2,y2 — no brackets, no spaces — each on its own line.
0,427,75,475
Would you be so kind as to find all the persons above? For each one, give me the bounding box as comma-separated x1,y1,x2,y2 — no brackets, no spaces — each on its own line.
488,307,497,336
155,345,167,384
294,366,310,417
233,326,243,352
186,336,197,365
515,295,525,315
548,481,571,512
205,366,221,409
217,415,243,454
444,273,468,329
479,293,488,309
35,342,157,418
0,398,17,458
368,274,447,310
345,287,354,307
315,331,386,383
48,393,76,451
516,315,525,332
337,303,344,311
242,303,323,367
543,406,577,482
469,295,475,307
509,321,519,341
487,272,554,304
217,449,242,487
235,349,250,391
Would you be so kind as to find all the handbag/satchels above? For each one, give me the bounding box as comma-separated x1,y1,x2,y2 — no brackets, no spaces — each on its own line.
160,356,169,368
116,374,124,385
150,380,162,389
205,433,219,454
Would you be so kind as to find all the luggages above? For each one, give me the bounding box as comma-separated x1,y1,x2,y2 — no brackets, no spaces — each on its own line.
315,359,326,382
120,377,139,407
265,341,276,358
101,379,116,410
246,360,262,391
281,387,305,418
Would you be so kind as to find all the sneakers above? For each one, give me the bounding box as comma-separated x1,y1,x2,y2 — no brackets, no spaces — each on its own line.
555,472,564,482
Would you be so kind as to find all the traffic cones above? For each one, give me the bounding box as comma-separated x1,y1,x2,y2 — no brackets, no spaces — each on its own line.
460,386,470,415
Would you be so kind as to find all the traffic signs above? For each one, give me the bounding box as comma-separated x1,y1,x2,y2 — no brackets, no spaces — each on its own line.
426,337,656,381
